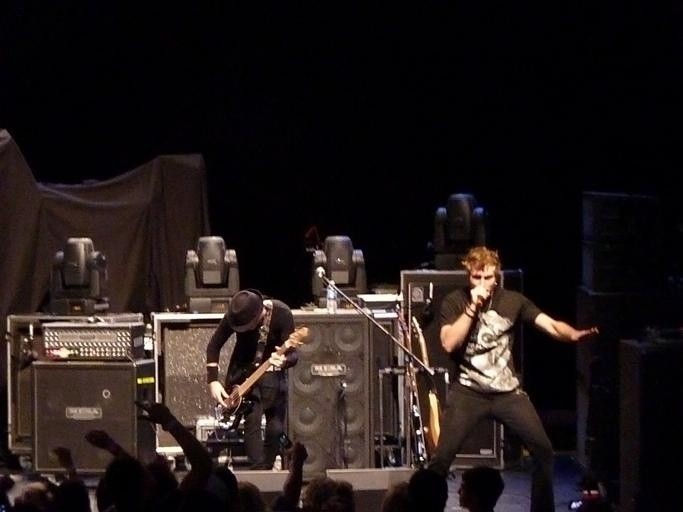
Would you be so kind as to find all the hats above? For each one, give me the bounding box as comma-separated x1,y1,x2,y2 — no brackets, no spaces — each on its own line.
225,288,265,334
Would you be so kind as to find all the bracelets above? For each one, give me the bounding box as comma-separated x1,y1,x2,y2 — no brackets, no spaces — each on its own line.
464,307,475,319
466,304,476,315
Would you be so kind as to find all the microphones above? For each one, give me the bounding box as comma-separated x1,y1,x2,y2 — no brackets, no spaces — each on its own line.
477,295,484,307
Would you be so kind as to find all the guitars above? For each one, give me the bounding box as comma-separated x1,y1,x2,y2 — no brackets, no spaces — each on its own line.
411,316,441,447
214,327,309,430
394,304,428,470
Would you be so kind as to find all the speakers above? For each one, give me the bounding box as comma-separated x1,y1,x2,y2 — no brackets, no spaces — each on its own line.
3,313,144,455
152,312,238,456
281,309,374,470
373,313,401,449
28,359,157,479
398,268,523,473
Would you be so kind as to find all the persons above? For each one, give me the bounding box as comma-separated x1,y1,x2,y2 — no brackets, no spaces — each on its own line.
423,243,603,511
457,466,505,512
205,287,299,471
1,397,449,511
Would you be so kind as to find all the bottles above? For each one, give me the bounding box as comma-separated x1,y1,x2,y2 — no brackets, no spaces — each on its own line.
326,282,338,315
